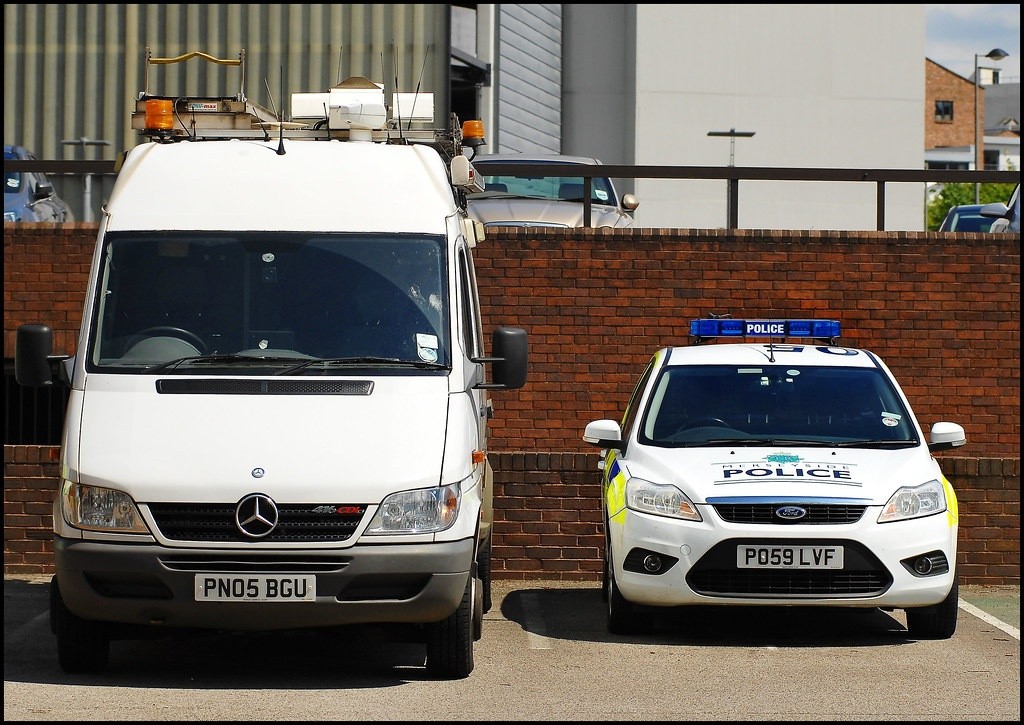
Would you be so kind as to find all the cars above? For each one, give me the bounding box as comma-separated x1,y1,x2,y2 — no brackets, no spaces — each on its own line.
3,144,74,226
584,317,980,649
939,203,1007,234
461,151,640,229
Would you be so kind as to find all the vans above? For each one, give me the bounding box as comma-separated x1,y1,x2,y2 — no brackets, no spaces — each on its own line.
11,134,531,680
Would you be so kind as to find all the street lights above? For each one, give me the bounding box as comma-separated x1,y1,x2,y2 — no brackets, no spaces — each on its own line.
975,47,1011,203
